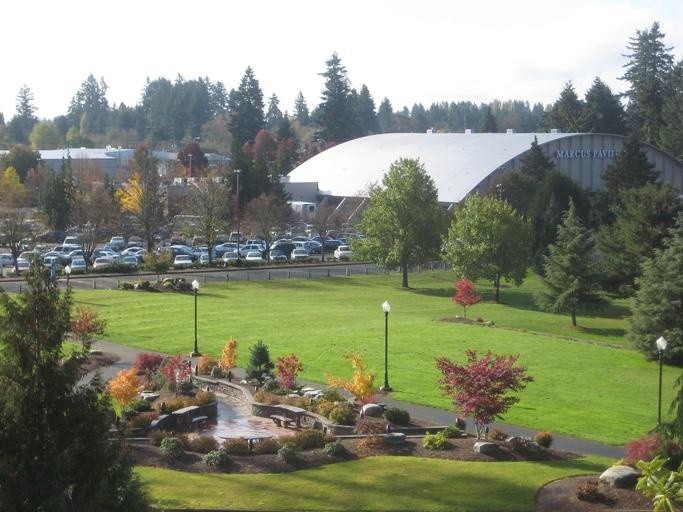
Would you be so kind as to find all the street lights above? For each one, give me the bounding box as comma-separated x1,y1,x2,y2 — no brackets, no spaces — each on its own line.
234,168,243,259
187,153,194,176
191,279,201,356
652,336,669,440
380,299,392,391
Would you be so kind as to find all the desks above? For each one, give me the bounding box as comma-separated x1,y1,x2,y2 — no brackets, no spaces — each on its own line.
272,404,307,427
171,405,199,433
219,434,272,446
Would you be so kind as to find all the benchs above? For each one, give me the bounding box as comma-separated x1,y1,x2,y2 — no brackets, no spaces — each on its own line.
270,414,294,429
192,415,209,429
